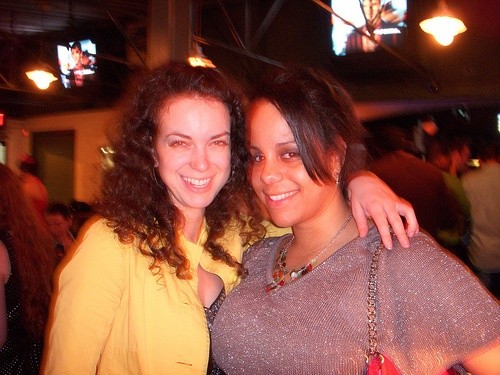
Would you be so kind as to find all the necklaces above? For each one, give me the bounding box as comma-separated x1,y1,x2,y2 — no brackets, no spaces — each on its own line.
265,213,352,292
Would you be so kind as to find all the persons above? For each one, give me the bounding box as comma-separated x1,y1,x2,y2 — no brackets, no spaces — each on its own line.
41,61,420,375
65,41,98,88
0,161,97,375
346,1,407,56
211,69,500,375
371,118,499,302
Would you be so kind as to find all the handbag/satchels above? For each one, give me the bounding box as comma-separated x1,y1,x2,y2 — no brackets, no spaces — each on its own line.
365,231,468,375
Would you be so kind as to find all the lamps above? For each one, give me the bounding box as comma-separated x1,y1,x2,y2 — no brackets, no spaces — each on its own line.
419,0,467,46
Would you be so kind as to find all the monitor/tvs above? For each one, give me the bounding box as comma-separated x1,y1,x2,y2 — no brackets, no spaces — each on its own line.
55,29,127,90
328,0,420,65
